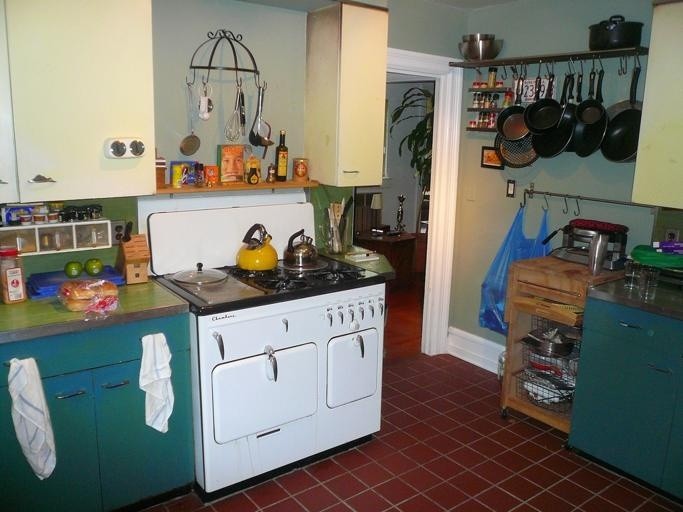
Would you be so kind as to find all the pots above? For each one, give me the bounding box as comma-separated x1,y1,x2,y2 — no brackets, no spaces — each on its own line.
494,68,642,168
588,16,642,51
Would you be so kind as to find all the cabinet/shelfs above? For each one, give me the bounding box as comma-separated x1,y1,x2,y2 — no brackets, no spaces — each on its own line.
1,0,157,205
463,86,507,132
565,296,681,506
0,214,112,258
304,0,391,188
624,0,681,215
1,314,197,510
496,255,627,437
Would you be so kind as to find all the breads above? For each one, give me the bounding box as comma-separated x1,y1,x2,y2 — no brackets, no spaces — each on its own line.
59,280,118,313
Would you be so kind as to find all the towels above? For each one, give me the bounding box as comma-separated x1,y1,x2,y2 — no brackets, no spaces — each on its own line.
139,330,175,435
9,357,55,481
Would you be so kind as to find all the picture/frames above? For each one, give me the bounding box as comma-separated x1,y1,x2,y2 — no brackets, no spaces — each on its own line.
480,145,505,170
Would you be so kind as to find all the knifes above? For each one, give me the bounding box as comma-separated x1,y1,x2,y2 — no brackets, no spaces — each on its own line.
122,220,133,242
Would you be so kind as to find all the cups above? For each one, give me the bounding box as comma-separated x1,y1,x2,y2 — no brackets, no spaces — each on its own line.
586,234,610,279
624,259,658,302
292,157,309,182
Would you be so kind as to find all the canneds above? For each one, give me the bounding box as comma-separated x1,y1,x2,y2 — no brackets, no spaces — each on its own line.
50,203,63,223
480,82,487,88
294,157,308,180
470,112,495,128
40,233,51,250
473,81,480,89
495,82,503,88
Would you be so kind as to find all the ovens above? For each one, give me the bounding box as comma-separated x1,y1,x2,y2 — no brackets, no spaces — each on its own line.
199,280,385,501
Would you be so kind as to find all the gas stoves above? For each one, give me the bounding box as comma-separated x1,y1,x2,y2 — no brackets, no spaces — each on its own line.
151,249,389,331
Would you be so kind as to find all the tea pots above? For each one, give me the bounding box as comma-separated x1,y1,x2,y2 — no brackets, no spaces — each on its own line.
237,225,279,272
283,230,321,268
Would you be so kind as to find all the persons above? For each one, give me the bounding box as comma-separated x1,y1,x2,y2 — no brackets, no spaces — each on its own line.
179,167,188,184
221,149,242,177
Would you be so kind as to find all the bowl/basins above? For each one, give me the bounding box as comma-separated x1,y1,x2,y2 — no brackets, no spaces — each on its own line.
533,342,573,358
458,39,503,63
462,34,494,41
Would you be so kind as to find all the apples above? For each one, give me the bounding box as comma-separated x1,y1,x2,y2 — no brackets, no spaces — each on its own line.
85,259,102,276
64,261,82,278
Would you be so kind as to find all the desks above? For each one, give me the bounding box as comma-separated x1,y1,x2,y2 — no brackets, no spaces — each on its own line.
354,227,417,291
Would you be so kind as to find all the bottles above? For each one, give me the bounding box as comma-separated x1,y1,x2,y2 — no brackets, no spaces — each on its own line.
168,161,215,190
468,67,512,130
20,204,101,253
243,128,288,184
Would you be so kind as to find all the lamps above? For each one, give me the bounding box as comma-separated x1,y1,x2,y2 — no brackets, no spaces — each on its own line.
354,192,372,234
368,193,382,237
